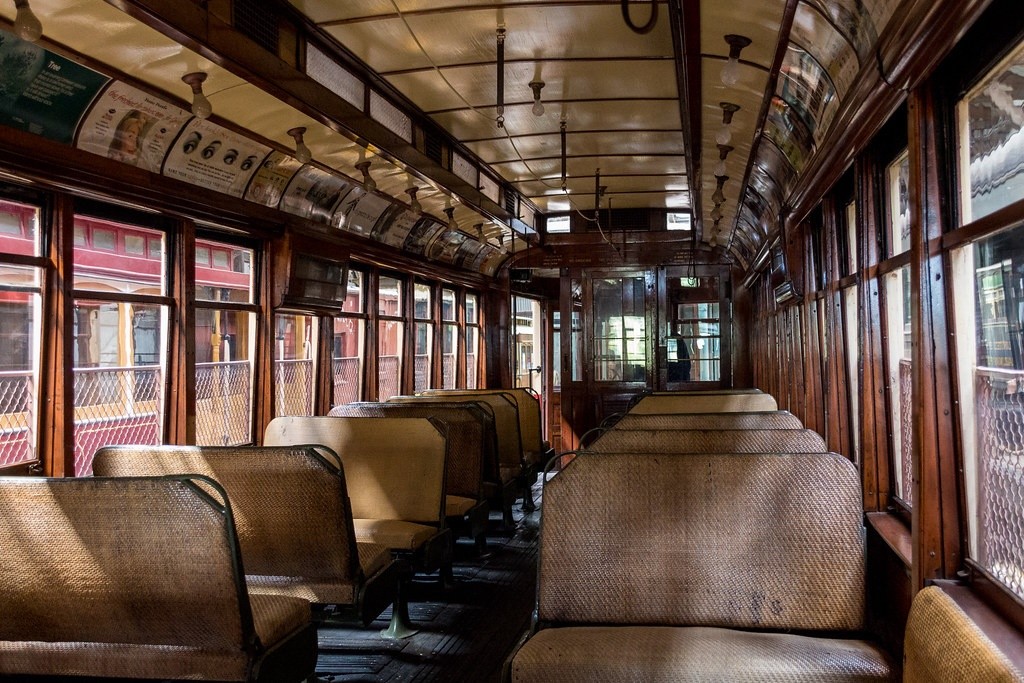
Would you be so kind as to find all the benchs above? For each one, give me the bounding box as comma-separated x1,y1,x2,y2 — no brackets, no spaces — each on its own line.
0,387,557,683
502,387,891,683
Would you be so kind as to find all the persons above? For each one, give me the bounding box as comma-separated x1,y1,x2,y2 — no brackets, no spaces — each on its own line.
669,332,691,382
109,119,141,165
